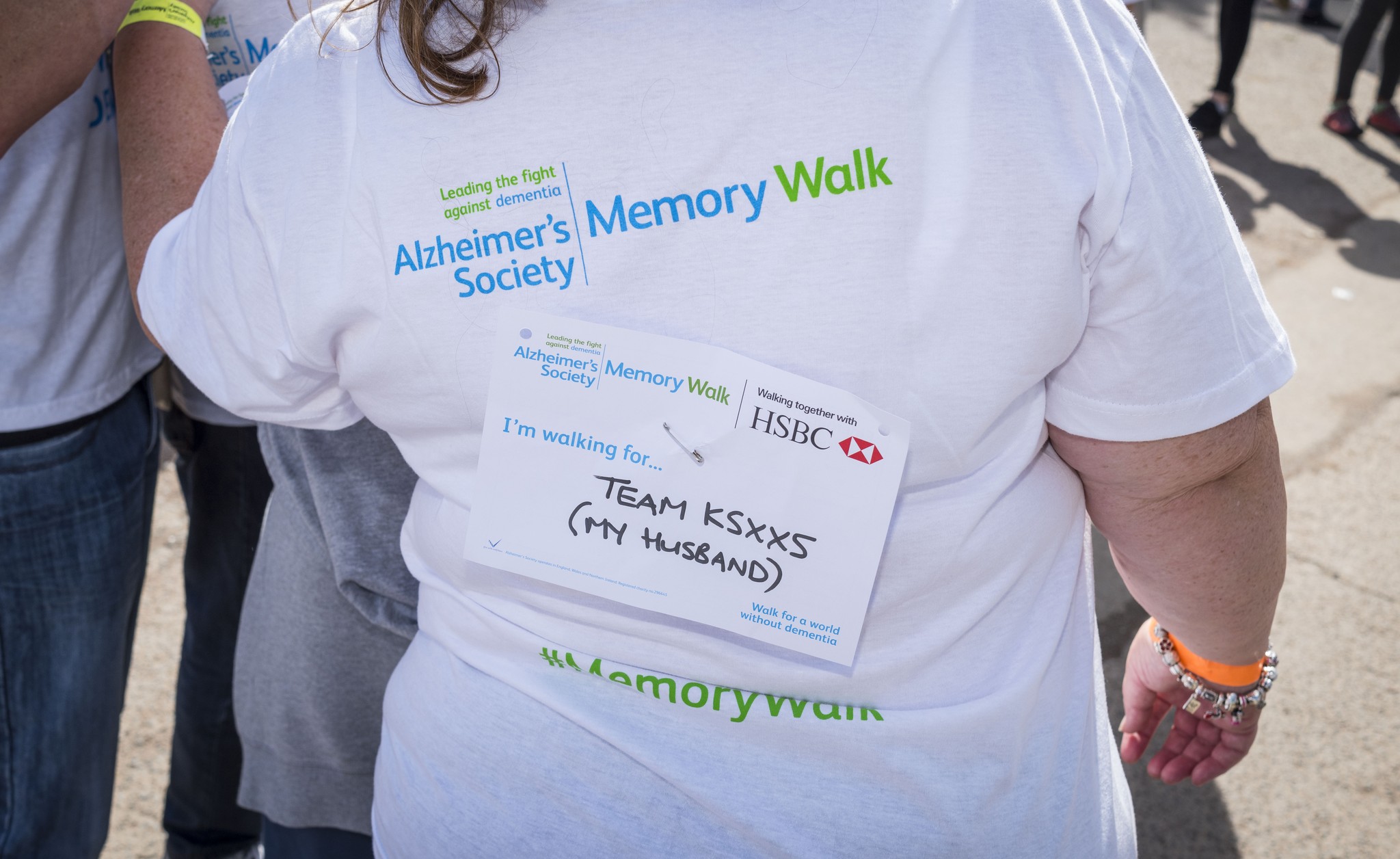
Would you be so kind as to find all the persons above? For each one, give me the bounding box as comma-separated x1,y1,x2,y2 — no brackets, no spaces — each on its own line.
1323,0,1400,137
1188,0,1255,140
1295,0,1344,32
0,1,421,858
108,0,1295,858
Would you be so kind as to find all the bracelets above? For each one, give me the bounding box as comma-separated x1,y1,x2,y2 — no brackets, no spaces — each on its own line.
1153,625,1279,725
1145,620,1270,686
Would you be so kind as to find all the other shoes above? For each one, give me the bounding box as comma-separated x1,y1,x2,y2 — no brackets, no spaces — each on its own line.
1189,87,1236,138
1367,99,1400,138
1323,103,1363,136
